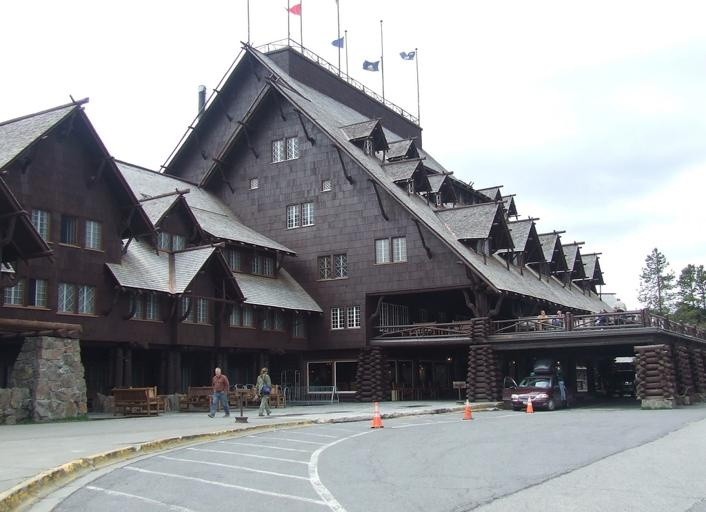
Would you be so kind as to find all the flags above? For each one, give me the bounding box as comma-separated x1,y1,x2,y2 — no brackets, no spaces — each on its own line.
330,36,344,49
284,4,301,16
400,51,415,61
362,59,380,72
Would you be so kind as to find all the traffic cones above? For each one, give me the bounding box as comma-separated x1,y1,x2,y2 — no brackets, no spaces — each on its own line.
371,402,383,428
462,400,473,420
527,395,533,413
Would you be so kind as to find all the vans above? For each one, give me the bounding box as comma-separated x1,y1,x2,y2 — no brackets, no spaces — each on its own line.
608,369,637,399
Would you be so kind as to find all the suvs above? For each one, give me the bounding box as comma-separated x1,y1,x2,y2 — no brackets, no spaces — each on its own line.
503,369,573,410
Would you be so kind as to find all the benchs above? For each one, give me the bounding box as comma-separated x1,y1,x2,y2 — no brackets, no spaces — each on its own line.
515,314,565,332
112,386,214,416
409,320,472,336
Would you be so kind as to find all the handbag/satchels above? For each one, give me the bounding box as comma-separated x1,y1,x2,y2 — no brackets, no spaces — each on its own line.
261,385,272,395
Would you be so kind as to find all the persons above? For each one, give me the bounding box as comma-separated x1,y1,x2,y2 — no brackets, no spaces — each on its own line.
538,310,550,330
256,367,272,416
207,367,230,418
598,308,609,330
552,310,563,330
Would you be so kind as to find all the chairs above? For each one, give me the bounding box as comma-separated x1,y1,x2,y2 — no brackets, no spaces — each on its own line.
228,384,289,408
